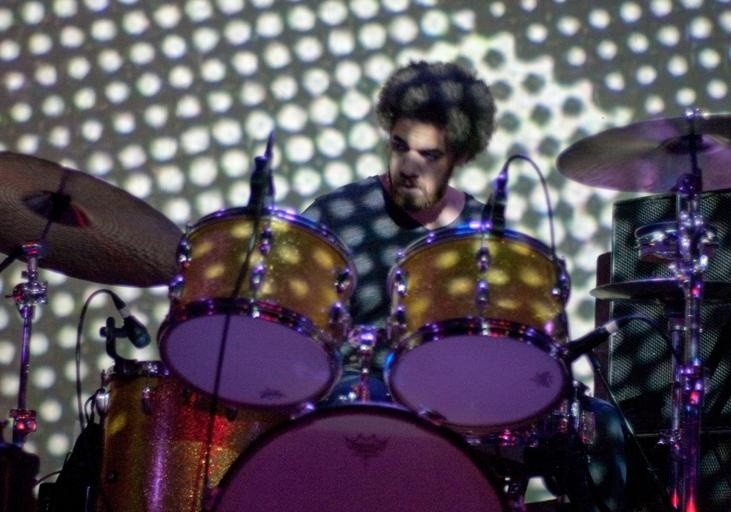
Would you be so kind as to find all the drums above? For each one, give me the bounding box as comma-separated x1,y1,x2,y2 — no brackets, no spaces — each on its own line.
155,206,358,408
95,358,286,507
207,399,524,508
382,223,575,433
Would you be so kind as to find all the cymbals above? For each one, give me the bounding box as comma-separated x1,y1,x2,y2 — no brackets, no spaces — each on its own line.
589,279,730,308
557,113,729,194
0,151,185,287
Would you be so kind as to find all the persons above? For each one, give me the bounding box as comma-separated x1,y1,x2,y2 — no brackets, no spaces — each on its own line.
299,59,498,382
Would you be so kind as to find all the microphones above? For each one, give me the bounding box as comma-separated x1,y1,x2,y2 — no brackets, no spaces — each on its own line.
108,291,151,348
248,138,274,202
482,163,508,224
554,315,635,367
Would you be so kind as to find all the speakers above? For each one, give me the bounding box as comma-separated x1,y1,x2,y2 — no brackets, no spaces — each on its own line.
609,190,730,511
